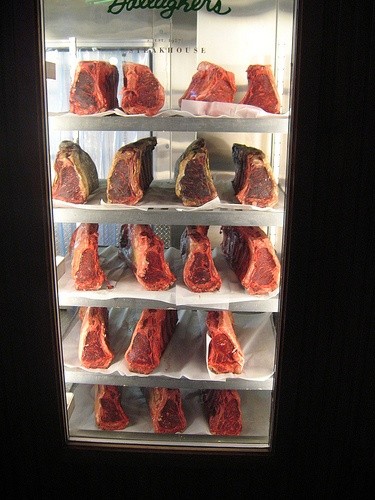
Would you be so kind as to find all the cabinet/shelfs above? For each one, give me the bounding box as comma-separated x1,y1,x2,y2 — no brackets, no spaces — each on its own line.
34,1,300,458
